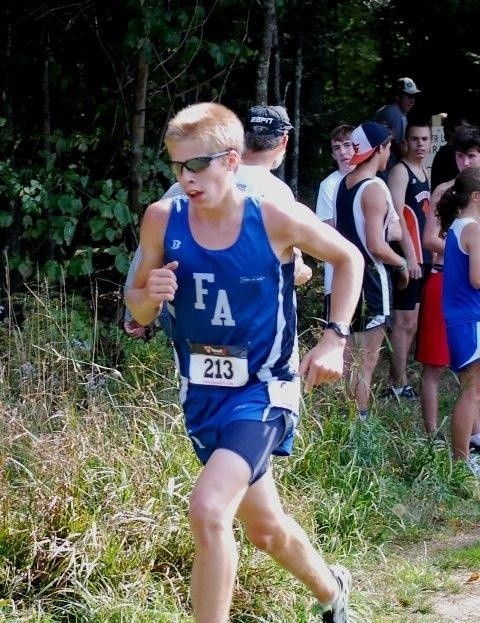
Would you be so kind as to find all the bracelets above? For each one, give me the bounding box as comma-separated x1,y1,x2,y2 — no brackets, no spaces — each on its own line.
399,259,407,271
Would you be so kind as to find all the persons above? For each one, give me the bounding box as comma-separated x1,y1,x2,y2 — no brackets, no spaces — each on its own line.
373,76,421,186
124,102,352,623
122,105,313,373
375,116,432,402
434,167,479,477
431,113,470,197
415,124,479,455
314,124,363,386
334,121,409,425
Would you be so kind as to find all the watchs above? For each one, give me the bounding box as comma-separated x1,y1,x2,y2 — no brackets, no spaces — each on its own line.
325,322,351,338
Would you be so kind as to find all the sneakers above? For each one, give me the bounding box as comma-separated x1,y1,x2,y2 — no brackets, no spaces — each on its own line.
322,565,353,622
376,384,421,401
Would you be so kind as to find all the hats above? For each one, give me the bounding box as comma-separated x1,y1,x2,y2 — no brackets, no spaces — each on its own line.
246,104,295,134
350,122,391,163
394,76,423,96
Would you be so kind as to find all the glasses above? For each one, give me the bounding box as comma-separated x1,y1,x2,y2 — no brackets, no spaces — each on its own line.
171,151,233,173
